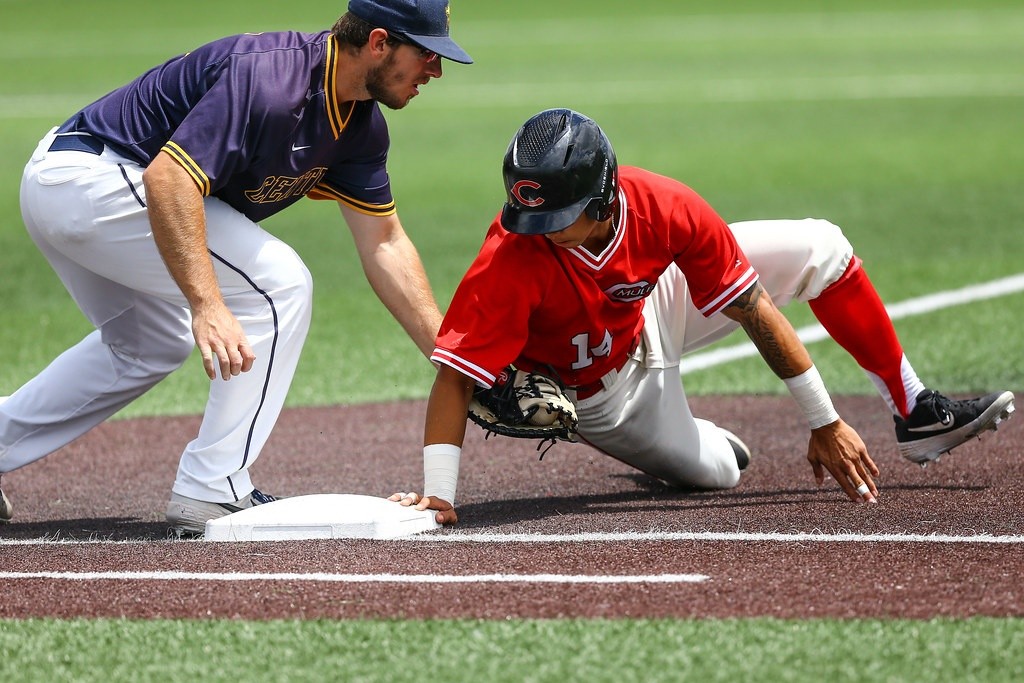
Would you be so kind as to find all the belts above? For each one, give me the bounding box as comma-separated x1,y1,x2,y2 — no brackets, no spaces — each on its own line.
577,349,635,401
48,135,104,156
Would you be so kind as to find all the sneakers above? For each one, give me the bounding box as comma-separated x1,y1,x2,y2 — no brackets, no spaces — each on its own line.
717,426,752,469
166,487,287,535
0,471,14,520
893,388,1016,469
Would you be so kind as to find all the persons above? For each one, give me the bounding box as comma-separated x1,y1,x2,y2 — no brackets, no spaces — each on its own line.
0,0,473,534
387,109,1014,523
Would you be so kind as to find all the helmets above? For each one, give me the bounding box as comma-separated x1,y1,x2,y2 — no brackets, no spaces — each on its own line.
500,108,620,235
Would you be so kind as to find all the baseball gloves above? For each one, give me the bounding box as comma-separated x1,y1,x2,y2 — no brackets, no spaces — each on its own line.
466,365,579,442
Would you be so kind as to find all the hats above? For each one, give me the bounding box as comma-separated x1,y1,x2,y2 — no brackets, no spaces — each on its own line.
348,0,474,64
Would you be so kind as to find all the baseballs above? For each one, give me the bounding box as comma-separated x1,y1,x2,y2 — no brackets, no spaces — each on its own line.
526,407,559,426
